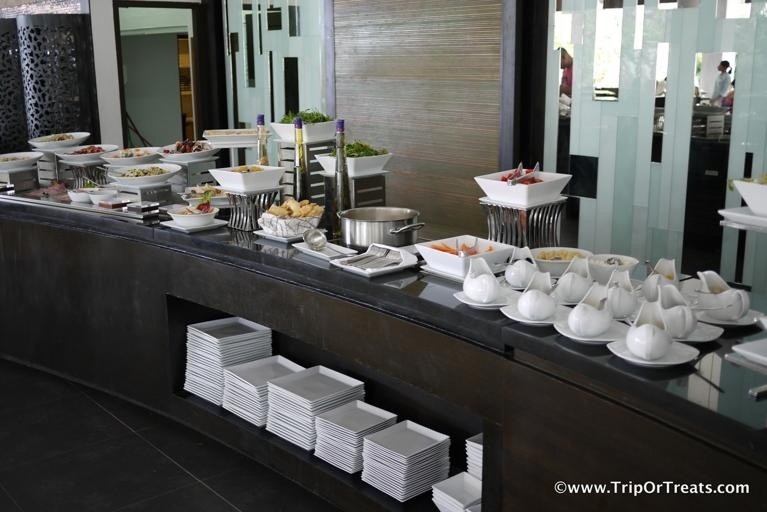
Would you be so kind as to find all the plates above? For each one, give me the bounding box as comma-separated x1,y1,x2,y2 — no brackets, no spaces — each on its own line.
291,242,359,262
717,206,767,228
452,277,767,369
160,219,229,234
183,315,483,512
253,230,327,244
0,132,221,186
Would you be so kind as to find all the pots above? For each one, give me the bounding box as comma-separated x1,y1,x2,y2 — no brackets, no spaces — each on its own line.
336,206,426,248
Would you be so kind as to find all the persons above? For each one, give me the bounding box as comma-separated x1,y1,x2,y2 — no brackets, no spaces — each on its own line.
709,60,734,106
557,47,572,98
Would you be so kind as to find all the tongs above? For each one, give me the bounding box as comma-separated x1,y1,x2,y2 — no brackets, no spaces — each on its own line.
507,161,539,185
455,237,478,257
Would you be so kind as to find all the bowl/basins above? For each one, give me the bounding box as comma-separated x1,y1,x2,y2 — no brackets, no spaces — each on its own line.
529,247,640,283
473,168,573,207
88,190,118,205
67,190,91,203
203,122,393,191
166,206,220,227
414,234,514,279
731,179,767,216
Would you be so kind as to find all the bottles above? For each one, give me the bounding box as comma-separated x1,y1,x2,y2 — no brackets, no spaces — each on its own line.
256,113,356,237
